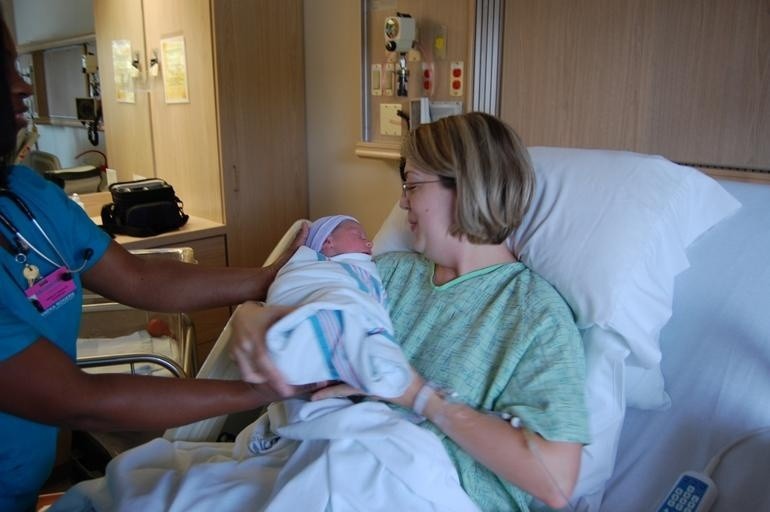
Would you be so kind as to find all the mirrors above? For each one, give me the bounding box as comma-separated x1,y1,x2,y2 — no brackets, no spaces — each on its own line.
0,0,165,218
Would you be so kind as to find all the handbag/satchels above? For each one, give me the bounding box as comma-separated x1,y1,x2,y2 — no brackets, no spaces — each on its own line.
101,178,188,237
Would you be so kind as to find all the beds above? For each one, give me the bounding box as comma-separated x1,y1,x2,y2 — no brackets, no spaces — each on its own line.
2,119,768,512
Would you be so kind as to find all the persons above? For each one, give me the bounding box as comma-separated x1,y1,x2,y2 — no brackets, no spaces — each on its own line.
0,15,344,512
227,112,594,512
265,214,413,399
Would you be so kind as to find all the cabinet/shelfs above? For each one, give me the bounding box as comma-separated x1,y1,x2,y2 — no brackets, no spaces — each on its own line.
211,1,309,336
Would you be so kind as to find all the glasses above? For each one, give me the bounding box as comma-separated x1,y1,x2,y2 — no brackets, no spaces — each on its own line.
401,179,443,193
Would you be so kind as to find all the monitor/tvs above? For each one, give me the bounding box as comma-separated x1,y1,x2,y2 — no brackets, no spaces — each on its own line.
74,97,102,121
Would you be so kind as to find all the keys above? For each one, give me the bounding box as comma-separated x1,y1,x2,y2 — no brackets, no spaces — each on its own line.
23,265,40,287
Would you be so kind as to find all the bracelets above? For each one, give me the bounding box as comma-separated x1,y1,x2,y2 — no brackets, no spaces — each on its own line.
413,382,435,415
317,381,327,388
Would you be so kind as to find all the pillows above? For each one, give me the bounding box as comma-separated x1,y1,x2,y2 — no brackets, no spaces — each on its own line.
459,141,733,411
